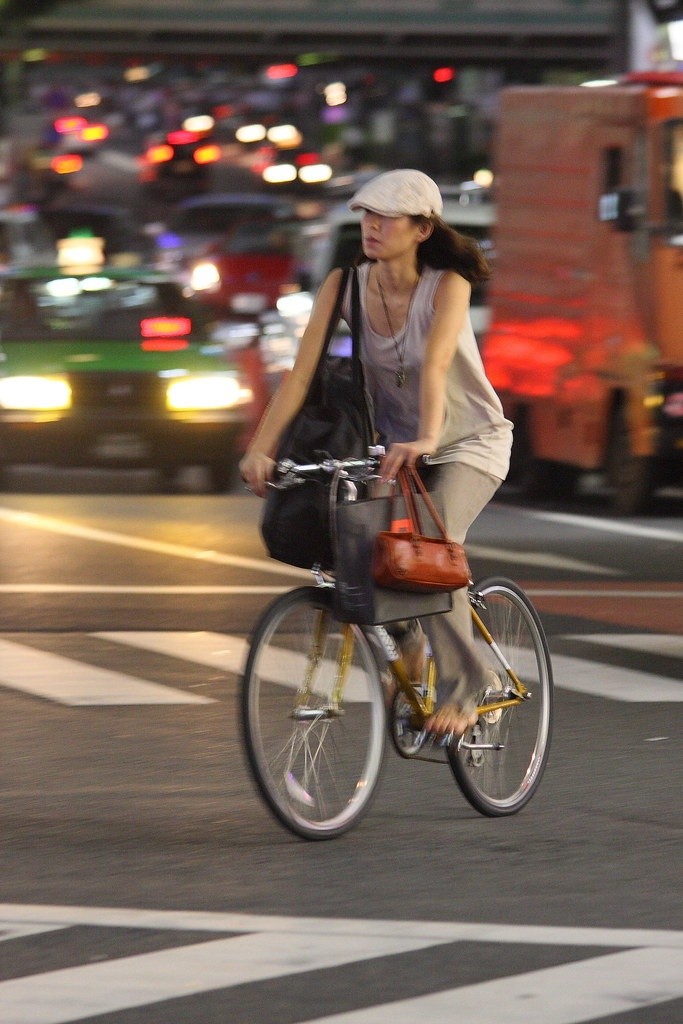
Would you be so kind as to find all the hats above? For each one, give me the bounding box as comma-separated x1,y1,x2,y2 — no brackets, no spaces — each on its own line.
347,169,443,218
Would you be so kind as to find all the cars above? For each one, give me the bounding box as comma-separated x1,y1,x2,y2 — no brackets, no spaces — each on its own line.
0,268,260,492
474,242,661,501
9,143,498,454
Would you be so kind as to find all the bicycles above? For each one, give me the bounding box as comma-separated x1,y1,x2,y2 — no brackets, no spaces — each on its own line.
240,450,554,844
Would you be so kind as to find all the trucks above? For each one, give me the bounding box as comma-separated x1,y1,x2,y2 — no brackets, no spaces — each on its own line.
486,76,683,458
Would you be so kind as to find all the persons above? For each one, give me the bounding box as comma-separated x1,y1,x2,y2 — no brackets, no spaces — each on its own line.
238,169,514,736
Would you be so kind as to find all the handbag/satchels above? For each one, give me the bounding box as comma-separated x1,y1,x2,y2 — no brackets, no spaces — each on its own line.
260,266,375,569
330,460,471,625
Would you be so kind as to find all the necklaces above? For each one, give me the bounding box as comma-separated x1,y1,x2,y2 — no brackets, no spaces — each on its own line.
375,272,419,389
